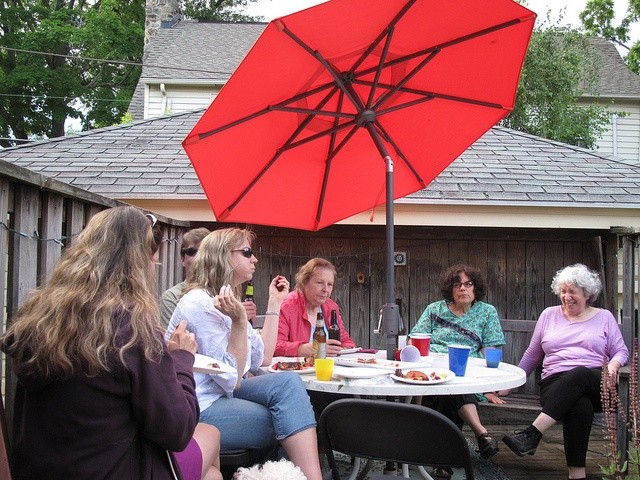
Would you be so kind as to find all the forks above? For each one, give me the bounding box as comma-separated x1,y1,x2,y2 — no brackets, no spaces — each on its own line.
228,263,243,284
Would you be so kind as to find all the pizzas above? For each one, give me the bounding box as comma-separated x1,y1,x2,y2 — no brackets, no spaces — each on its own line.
271,361,302,370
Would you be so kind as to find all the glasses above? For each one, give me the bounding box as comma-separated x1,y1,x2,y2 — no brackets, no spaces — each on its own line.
146,212,159,227
181,247,198,257
231,247,255,259
453,281,475,289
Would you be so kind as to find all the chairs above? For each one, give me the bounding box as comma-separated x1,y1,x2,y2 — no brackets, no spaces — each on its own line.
313,397,472,479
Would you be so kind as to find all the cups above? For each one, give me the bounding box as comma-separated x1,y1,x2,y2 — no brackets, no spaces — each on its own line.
410,336,430,356
486,348,502,368
448,344,471,377
314,358,334,380
396,345,420,363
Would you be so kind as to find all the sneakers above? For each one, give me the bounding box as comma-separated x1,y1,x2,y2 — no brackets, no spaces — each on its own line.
503,428,540,457
477,432,501,460
431,467,452,480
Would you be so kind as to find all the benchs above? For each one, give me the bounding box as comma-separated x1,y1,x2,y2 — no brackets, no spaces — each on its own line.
473,316,550,415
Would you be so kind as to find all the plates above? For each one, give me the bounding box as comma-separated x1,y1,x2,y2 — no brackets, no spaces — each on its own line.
335,368,384,378
341,347,362,354
391,368,455,384
383,362,426,369
337,358,388,367
268,360,315,373
193,353,237,375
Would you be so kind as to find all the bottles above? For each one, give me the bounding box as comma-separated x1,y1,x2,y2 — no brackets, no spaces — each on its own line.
329,310,340,356
242,282,254,327
313,313,326,359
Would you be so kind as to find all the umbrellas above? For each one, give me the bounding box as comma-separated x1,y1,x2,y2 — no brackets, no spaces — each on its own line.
181,0,537,360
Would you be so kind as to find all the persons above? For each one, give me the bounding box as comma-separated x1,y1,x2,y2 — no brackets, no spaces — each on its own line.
498,262,630,480
161,226,323,480
158,227,211,332
406,264,507,480
273,256,357,357
1,205,224,480
